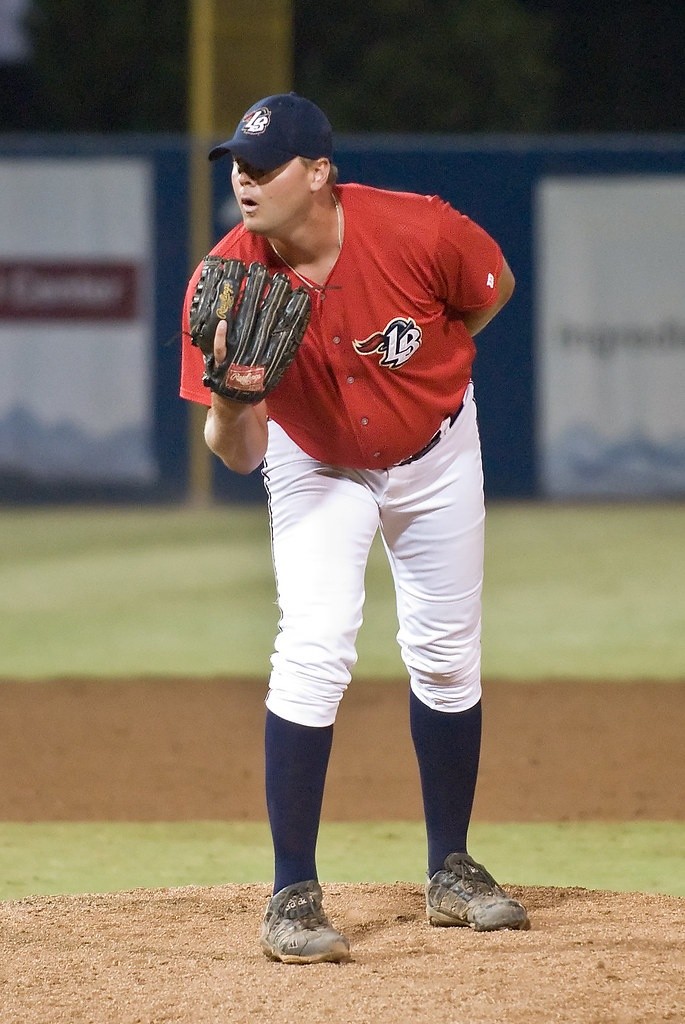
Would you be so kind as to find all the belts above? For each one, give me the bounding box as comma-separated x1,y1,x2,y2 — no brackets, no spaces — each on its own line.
393,401,464,466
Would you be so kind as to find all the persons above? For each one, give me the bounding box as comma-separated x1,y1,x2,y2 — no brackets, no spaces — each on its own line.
178,93,534,965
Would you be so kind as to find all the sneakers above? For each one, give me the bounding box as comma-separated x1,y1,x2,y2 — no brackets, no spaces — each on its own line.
425,852,527,931
260,880,351,963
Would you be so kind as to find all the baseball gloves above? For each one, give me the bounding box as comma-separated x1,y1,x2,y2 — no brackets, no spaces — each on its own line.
188,253,312,405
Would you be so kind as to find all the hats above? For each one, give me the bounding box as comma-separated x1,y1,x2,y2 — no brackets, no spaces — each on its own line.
208,91,333,170
